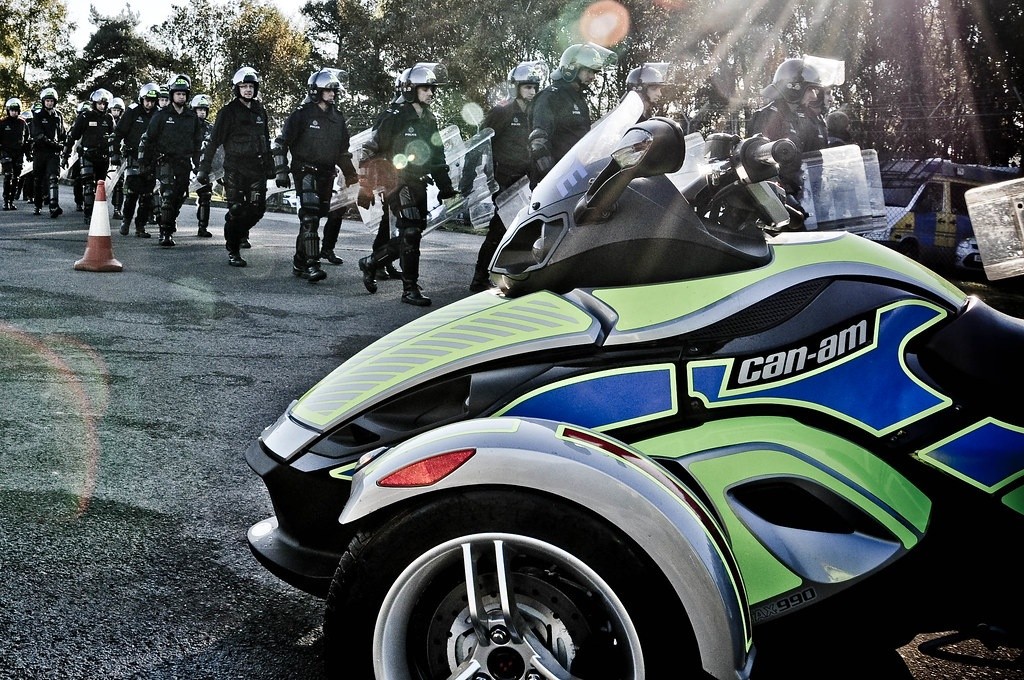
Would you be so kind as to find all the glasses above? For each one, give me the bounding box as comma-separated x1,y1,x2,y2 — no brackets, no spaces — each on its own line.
237,84,255,89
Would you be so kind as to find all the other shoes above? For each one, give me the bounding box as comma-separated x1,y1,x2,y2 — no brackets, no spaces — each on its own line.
2,191,433,307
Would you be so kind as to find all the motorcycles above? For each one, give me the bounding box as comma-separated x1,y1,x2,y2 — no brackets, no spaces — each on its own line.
242,84,1024,679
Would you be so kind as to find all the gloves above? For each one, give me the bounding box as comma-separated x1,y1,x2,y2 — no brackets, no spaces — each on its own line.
0,146,473,210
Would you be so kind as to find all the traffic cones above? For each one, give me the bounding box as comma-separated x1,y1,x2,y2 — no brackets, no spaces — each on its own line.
72,180,125,274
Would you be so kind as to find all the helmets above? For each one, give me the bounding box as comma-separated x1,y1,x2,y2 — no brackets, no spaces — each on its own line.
42,89,58,107
156,86,171,107
303,72,341,104
390,71,403,105
800,85,832,110
138,86,160,108
760,59,821,105
168,77,190,102
6,98,21,117
626,67,665,96
77,101,92,117
233,70,259,98
396,65,438,105
31,102,43,116
21,111,33,125
107,100,124,119
549,44,601,84
90,92,109,112
191,96,209,118
506,65,541,98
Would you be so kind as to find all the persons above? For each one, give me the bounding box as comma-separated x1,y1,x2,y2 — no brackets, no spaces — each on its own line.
526,42,617,191
0,63,457,305
748,52,852,232
460,59,550,294
625,62,671,125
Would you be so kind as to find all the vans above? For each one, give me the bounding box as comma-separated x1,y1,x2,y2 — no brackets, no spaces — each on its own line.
837,156,1024,277
